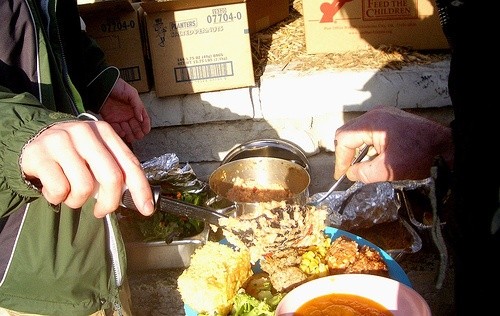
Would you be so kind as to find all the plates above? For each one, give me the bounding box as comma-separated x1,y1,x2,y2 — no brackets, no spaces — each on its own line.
275,274,432,316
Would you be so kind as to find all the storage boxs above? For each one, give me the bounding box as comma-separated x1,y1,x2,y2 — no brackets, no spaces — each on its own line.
78,0,449,99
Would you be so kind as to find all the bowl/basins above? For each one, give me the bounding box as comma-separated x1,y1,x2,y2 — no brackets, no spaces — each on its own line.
203,157,311,246
183,225,411,316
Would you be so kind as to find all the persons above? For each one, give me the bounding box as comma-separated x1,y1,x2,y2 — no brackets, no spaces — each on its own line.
333,0,500,316
0,0,156,316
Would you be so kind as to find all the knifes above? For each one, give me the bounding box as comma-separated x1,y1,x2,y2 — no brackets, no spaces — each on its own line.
92,182,229,231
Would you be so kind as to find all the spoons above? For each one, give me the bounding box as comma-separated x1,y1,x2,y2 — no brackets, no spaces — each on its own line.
303,145,371,207
